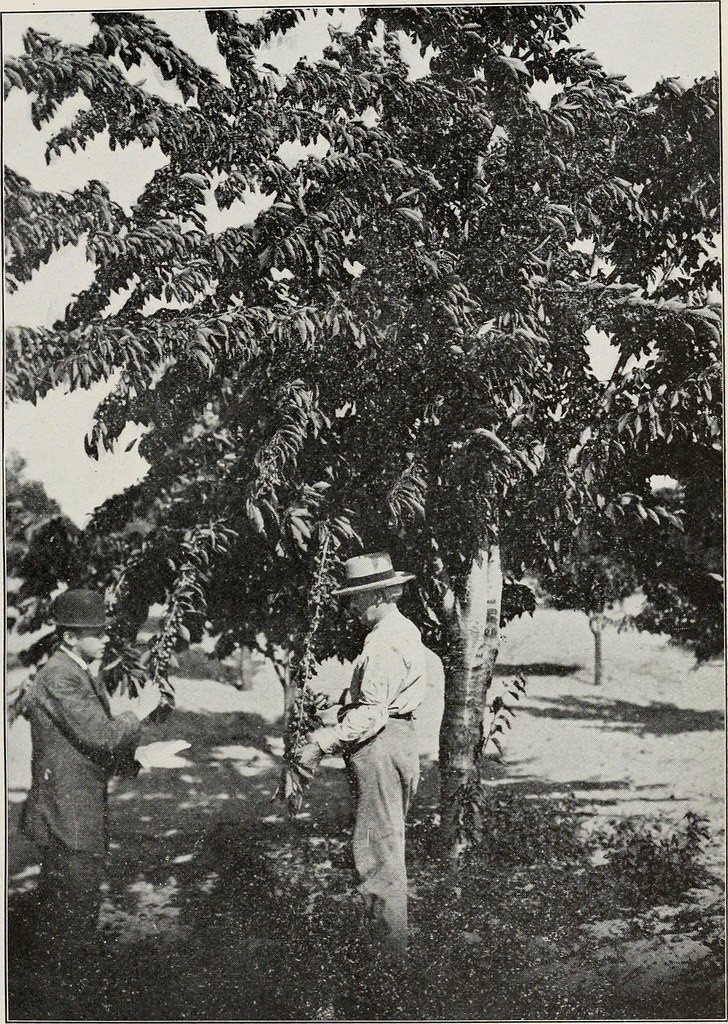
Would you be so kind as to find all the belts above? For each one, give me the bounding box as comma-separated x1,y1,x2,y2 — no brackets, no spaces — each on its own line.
390,712,411,721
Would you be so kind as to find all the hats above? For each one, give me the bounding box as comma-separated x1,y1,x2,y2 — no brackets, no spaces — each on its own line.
331,550,416,596
48,589,109,628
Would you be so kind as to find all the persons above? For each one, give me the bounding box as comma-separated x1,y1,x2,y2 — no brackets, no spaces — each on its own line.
16,590,161,1018
293,551,425,990
